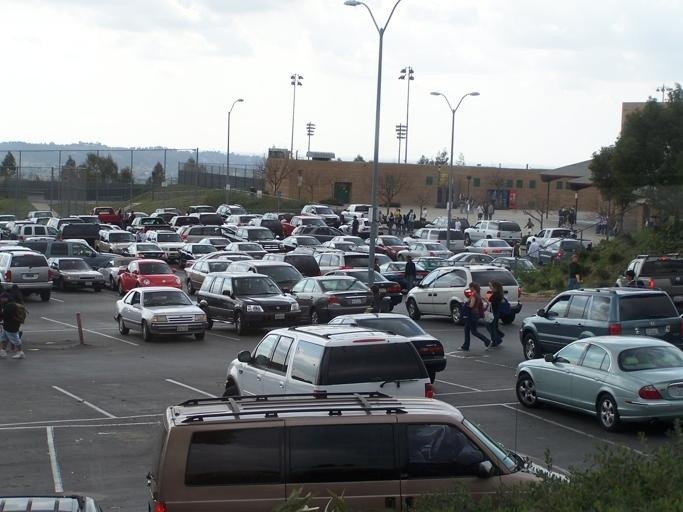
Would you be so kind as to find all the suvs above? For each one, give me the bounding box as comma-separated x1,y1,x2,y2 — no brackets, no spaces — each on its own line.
148,391,574,512
314,251,381,273
615,254,683,303
197,272,301,335
0,249,53,300
225,326,433,412
520,287,683,359
464,220,522,247
425,216,469,229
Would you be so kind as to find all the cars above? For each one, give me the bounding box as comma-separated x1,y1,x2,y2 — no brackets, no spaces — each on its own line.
320,270,403,313
1,209,102,240
224,259,304,293
323,313,447,383
515,335,683,430
491,257,537,275
376,262,431,292
47,258,106,292
526,228,577,254
406,264,522,324
465,239,515,258
183,259,232,294
539,238,580,265
91,203,411,260
447,252,495,265
113,287,208,340
98,260,140,289
118,260,182,298
409,241,453,263
283,276,374,327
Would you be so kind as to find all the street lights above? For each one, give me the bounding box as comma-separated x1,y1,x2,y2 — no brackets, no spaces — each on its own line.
394,121,407,164
399,66,414,164
224,98,244,206
342,0,404,287
429,92,480,250
307,123,315,160
289,76,301,160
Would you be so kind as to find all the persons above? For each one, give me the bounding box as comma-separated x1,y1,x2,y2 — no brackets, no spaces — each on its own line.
644,209,682,231
0,285,26,305
528,238,540,262
404,256,416,290
593,210,626,236
557,202,580,227
421,208,428,228
0,293,29,359
488,281,510,346
457,283,494,351
375,257,380,283
281,214,288,222
115,208,157,246
359,214,365,235
625,270,638,287
352,216,359,235
523,218,534,234
568,254,583,289
375,204,417,238
513,239,522,257
446,192,497,231
340,209,350,224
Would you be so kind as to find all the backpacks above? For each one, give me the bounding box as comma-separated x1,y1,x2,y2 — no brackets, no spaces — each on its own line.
11,301,27,323
497,294,511,318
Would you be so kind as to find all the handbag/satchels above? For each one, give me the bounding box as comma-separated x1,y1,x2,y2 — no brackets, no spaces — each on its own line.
479,298,485,318
496,296,510,314
483,304,494,323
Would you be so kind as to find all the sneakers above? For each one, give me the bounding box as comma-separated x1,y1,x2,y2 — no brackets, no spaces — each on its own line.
11,353,25,359
498,341,504,347
489,345,497,349
485,341,492,352
457,346,469,352
0,350,8,359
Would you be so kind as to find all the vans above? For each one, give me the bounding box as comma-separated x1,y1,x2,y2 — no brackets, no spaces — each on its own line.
0,239,102,259
0,493,103,512
261,253,320,283
414,227,466,254
